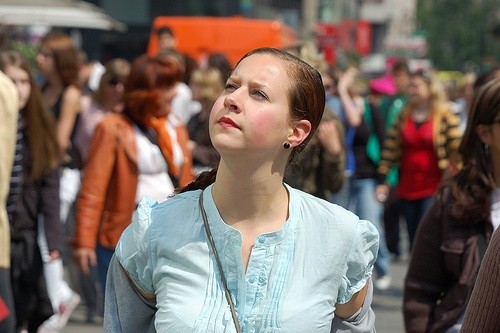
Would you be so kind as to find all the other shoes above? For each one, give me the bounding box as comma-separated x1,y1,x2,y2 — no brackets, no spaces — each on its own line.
376,274,391,290
